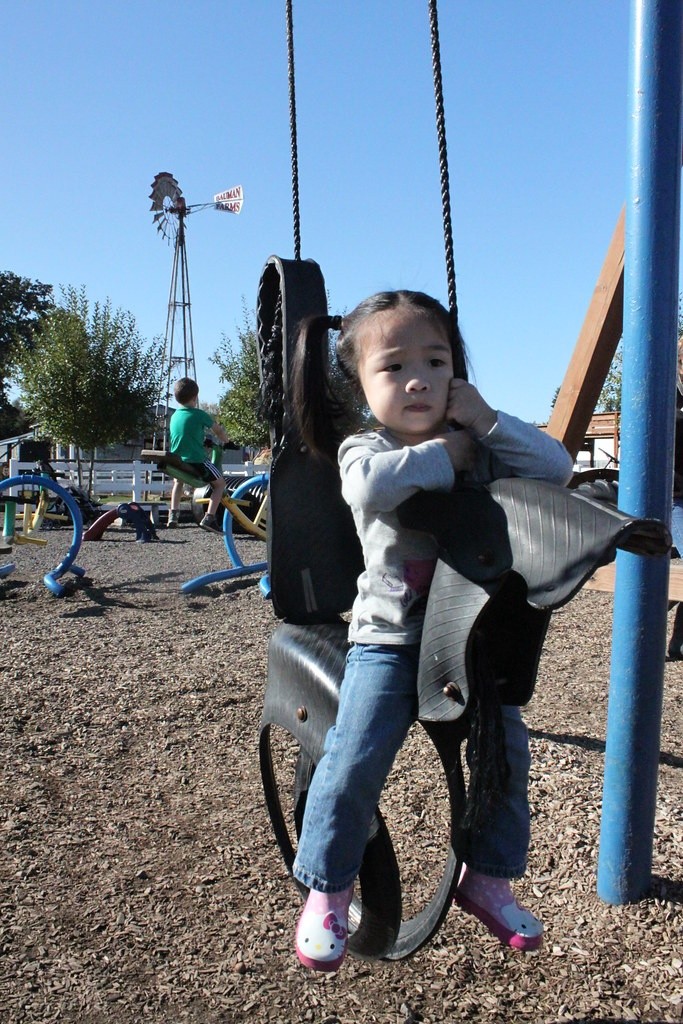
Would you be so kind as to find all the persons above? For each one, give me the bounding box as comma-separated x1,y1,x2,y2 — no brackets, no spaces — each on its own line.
167,378,228,536
293,289,574,972
672,338,683,562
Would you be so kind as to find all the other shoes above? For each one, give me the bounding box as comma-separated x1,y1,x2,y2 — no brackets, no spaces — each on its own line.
166,509,180,528
199,512,223,534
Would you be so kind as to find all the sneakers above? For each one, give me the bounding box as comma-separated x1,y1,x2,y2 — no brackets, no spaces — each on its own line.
452,861,544,950
294,883,354,972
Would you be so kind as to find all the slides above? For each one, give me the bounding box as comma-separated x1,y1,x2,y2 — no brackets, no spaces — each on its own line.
83,506,118,540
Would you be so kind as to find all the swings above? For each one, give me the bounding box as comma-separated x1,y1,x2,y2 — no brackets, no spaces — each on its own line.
248,0,676,961
